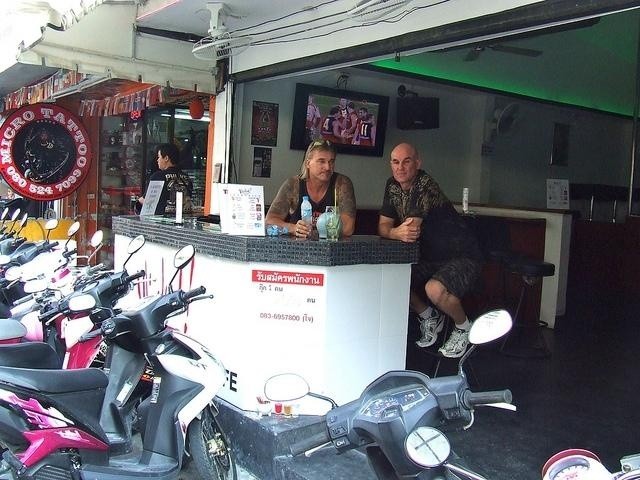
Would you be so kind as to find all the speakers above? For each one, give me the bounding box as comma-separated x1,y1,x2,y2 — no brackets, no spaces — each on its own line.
395,94,441,132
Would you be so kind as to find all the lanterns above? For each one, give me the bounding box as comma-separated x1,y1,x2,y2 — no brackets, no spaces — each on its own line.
190,97,204,119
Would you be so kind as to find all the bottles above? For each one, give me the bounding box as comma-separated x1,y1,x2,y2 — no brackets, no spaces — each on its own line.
316,212,328,238
104,118,165,146
300,196,313,227
108,150,121,170
263,223,287,237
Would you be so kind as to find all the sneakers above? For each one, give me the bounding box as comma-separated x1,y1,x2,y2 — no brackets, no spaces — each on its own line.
437,320,475,360
414,309,446,353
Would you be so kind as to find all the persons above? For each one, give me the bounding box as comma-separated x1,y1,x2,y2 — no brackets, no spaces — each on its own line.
134,145,193,215
379,143,487,358
305,95,376,146
29,130,57,154
265,139,356,238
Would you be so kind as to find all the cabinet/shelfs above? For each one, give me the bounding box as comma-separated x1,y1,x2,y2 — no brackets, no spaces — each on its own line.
103,135,160,196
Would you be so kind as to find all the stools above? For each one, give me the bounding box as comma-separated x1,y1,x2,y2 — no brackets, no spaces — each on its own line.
417,280,487,390
496,259,555,358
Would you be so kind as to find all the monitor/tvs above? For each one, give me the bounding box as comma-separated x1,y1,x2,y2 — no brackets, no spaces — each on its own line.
288,81,390,158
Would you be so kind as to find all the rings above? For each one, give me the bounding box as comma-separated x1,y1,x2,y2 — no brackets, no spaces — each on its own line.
296,232,299,237
296,228,299,232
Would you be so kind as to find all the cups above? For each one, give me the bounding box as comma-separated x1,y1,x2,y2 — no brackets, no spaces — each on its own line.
325,205,342,242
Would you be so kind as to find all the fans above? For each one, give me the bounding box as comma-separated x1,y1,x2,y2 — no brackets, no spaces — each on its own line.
483,99,522,143
434,43,544,62
190,4,250,61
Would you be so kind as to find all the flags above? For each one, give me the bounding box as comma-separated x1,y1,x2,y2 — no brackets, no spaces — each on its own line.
6,71,176,117
